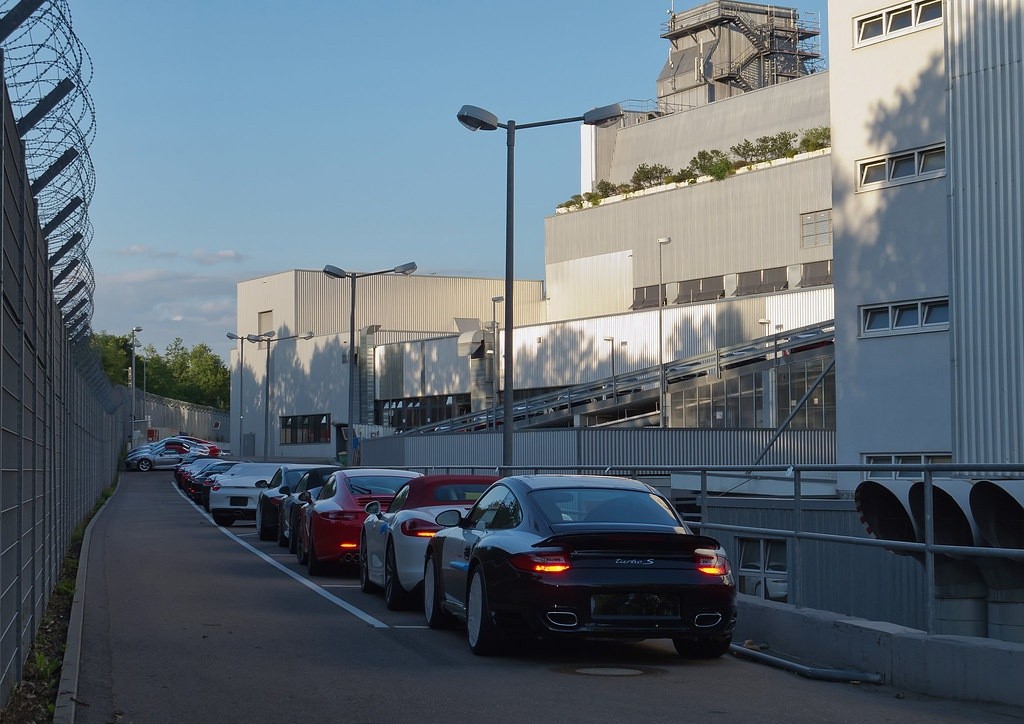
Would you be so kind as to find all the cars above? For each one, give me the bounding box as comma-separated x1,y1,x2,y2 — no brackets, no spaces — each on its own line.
667,363,707,384
278,467,354,556
295,469,425,577
739,562,787,600
465,378,641,431
359,474,506,612
124,435,302,528
422,474,738,664
255,465,338,543
782,330,833,356
720,348,766,371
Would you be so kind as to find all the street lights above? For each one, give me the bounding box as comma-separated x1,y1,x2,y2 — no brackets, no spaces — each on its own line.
132,326,142,450
226,331,275,456
457,105,624,480
759,319,770,360
323,261,418,467
657,237,671,428
603,337,617,402
247,332,314,463
491,296,505,431
